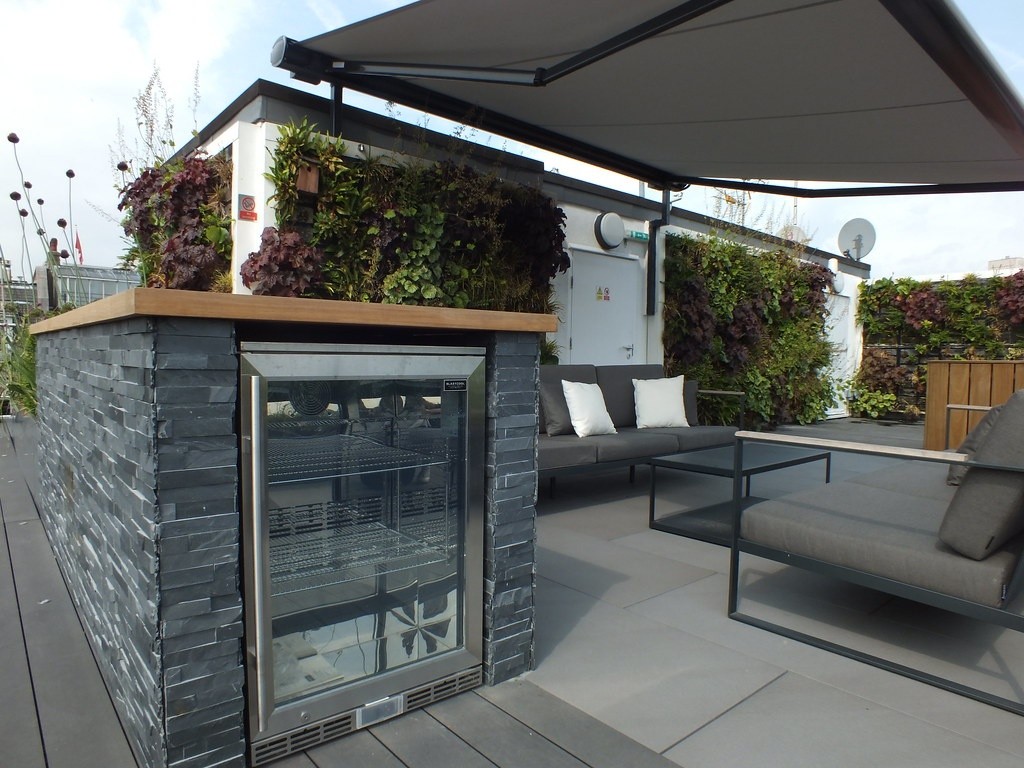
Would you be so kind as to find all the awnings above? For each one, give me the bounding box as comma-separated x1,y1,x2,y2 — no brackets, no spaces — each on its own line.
269,0,1022,230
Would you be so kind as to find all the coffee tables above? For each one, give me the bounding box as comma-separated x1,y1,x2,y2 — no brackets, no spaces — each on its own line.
649,442,831,547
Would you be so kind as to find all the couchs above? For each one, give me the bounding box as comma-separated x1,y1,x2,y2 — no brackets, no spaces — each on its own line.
538,363,745,500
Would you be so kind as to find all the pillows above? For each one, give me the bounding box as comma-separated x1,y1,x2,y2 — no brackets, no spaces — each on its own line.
947,404,1004,487
540,380,575,436
561,379,618,438
632,375,690,429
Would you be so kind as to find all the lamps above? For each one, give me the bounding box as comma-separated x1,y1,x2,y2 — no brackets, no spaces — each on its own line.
830,271,845,293
594,211,624,250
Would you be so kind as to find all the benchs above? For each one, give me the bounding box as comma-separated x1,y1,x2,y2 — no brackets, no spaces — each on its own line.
728,386,1024,718
